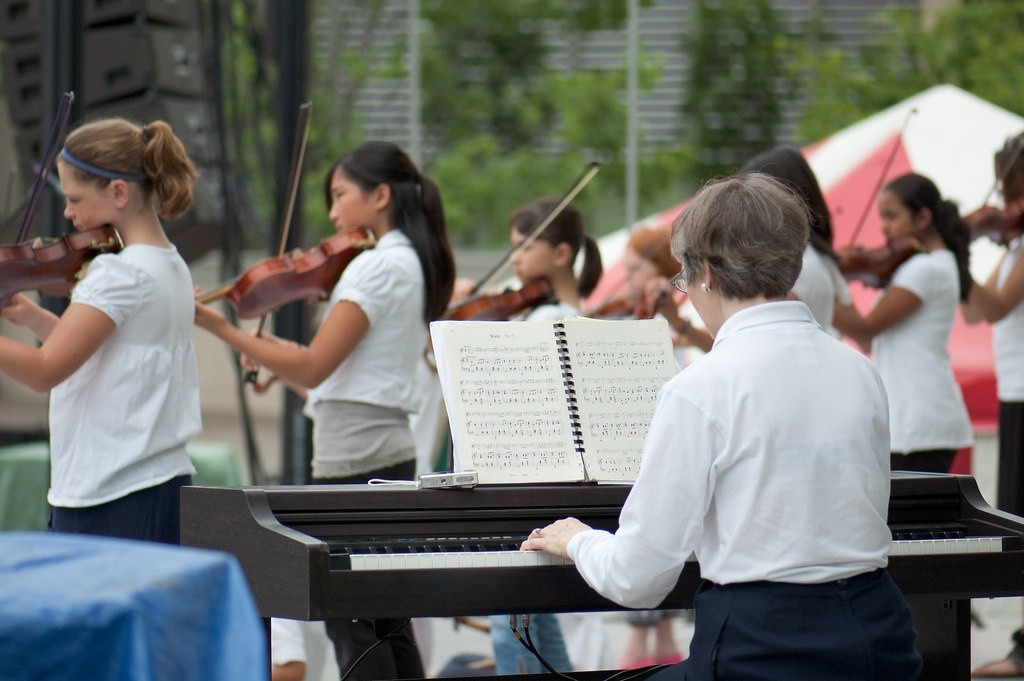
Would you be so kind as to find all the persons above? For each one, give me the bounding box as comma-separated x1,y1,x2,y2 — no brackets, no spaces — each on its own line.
519,175,932,681
615,225,716,666
970,131,1024,679
0,117,202,549
442,199,604,680
834,172,977,473
736,144,833,340
195,141,458,681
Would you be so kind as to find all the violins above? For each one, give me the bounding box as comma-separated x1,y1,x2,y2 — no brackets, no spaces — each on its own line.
959,200,1024,246
427,276,555,321
833,240,926,278
0,224,123,305
195,225,375,319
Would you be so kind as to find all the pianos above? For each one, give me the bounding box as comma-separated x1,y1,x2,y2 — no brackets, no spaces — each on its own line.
180,470,1023,620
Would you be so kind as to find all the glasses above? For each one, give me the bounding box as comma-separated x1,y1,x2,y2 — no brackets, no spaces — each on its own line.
670,265,689,294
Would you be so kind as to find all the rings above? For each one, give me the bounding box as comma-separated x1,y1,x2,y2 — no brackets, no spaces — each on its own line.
535,528,542,538
